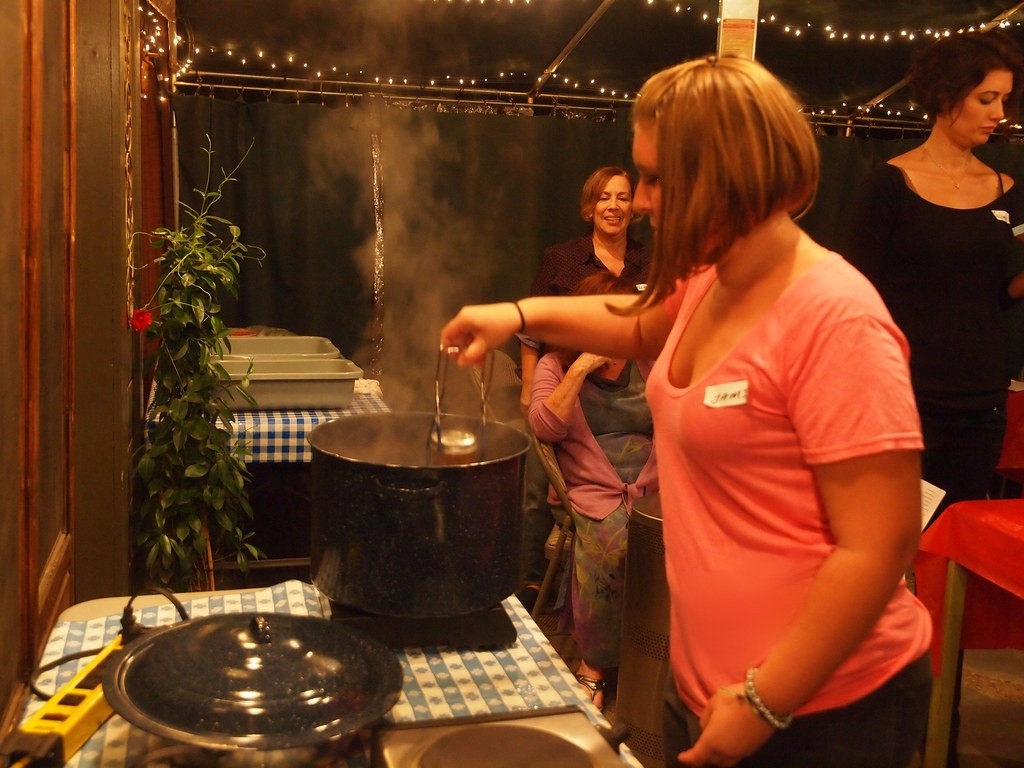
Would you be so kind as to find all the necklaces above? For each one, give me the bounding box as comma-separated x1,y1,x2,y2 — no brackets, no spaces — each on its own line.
921,144,974,190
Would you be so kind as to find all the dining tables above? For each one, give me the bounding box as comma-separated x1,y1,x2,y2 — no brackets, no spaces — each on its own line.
5,580,644,768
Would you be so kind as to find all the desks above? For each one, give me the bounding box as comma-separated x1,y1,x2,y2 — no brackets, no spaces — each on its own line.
191,379,394,573
920,497,1024,767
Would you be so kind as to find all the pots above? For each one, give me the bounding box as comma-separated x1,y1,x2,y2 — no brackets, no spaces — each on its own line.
308,410,532,620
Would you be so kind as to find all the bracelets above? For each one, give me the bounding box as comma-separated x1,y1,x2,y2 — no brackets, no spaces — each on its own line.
512,302,525,334
742,668,794,730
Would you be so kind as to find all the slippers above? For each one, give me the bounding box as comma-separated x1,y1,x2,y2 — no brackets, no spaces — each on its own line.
576,674,607,712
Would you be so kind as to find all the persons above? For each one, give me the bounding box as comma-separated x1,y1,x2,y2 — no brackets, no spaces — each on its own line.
442,58,934,768
519,167,652,606
865,36,1024,519
527,272,658,714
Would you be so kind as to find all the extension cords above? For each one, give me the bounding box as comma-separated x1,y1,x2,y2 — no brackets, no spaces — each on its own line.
0,628,157,768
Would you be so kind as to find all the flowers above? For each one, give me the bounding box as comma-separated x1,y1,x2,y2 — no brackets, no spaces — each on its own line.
129,308,163,333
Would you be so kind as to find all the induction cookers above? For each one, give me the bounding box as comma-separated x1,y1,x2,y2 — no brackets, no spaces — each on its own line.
374,712,633,768
330,600,520,648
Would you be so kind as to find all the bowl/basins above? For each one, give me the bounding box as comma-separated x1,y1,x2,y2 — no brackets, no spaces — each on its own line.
203,336,359,412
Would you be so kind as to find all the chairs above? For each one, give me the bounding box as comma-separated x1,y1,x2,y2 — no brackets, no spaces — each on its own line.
529,430,578,638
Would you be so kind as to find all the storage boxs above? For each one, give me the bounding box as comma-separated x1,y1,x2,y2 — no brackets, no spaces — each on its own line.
210,335,341,360
206,358,363,413
